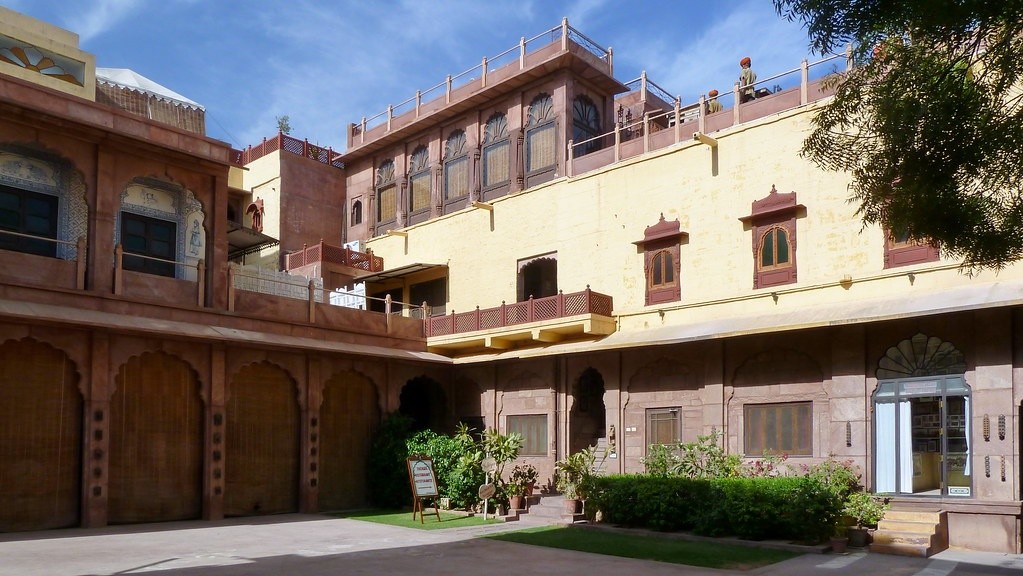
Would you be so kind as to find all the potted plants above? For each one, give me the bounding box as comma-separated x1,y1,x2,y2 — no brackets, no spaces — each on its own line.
562,484,581,512
511,462,539,494
503,484,525,508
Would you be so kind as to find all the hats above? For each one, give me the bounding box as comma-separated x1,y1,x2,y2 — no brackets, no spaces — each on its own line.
740,57,751,68
709,90,718,97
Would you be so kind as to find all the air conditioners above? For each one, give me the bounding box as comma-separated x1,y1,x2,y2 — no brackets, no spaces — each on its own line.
343,240,366,254
845,489,886,547
829,521,848,553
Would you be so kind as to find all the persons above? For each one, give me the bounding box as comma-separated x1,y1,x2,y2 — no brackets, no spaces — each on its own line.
707,90,723,115
740,57,757,103
